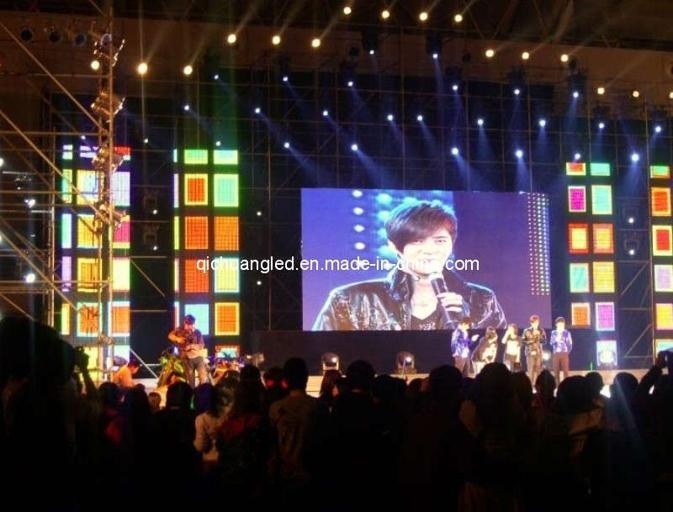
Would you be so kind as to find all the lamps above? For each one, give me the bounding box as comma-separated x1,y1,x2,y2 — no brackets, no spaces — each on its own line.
89,9,129,235
393,351,416,377
320,351,340,377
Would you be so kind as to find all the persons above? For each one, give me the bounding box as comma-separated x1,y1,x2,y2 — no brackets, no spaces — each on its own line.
312,198,508,330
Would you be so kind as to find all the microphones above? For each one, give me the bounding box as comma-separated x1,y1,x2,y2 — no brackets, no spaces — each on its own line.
429,272,461,329
